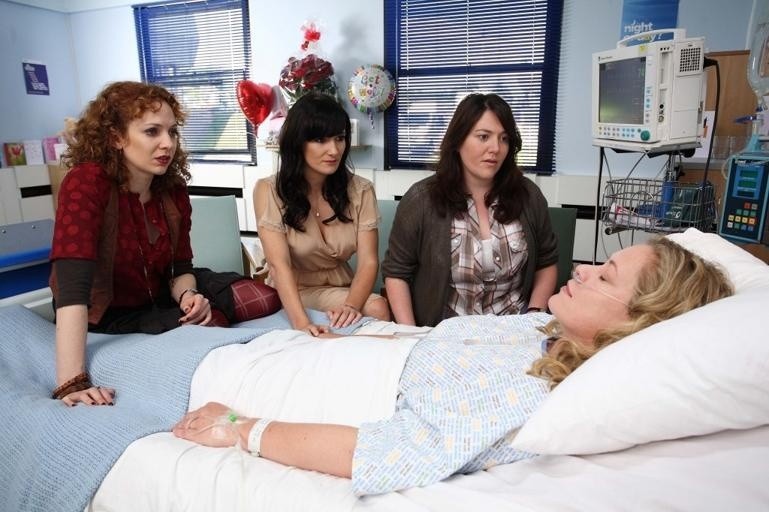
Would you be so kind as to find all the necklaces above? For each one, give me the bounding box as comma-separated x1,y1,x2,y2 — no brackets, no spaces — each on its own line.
310,188,320,217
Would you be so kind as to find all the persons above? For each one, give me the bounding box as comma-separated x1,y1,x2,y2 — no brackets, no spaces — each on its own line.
49,81,282,407
171,240,734,499
380,93,561,326
253,92,390,336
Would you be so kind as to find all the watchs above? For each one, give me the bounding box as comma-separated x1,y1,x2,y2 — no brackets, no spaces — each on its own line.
178,288,199,306
527,307,547,313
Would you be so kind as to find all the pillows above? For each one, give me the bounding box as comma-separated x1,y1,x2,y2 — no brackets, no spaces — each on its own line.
508,226,768,456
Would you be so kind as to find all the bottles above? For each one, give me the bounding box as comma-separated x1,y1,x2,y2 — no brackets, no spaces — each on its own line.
747,22,769,103
659,171,673,221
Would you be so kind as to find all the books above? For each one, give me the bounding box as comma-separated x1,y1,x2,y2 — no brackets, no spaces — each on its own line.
4,137,68,167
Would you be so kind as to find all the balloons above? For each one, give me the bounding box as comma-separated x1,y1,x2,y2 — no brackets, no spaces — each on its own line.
347,63,397,132
236,80,275,138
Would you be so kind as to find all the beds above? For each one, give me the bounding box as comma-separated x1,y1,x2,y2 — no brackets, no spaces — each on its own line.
1,274,769,510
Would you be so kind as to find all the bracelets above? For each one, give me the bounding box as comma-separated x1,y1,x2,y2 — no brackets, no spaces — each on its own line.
51,372,94,400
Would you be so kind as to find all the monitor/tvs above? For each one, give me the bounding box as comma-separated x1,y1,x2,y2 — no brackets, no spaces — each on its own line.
591,26,708,151
